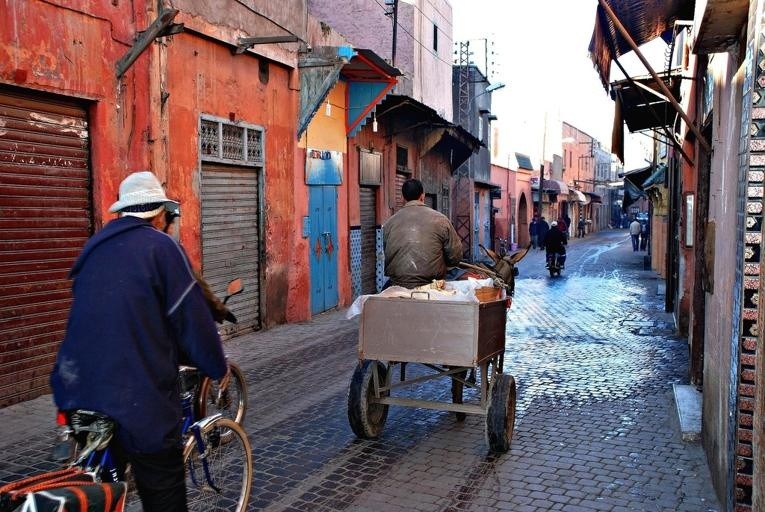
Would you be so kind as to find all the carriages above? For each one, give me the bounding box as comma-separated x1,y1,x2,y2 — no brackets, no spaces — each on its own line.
345,240,533,453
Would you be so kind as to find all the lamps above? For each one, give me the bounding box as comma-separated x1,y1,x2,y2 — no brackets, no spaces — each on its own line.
325,99,331,117
373,113,378,132
469,82,506,113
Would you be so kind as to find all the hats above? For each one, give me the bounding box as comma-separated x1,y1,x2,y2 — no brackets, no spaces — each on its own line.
107,171,180,220
551,221,558,227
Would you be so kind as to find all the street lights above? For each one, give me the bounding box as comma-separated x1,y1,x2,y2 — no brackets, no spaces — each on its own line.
536,135,575,222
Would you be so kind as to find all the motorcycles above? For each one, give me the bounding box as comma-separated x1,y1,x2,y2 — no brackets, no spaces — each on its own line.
496,236,510,258
50,273,252,479
545,241,565,278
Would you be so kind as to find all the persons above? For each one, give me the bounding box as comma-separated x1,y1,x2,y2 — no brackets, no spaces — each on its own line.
565,214,571,230
163,209,239,325
578,218,586,238
543,220,567,269
640,219,649,251
379,179,460,292
49,169,233,512
529,217,538,250
629,218,641,251
538,217,550,251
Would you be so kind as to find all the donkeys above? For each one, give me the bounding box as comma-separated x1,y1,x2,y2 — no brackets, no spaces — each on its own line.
450,244,532,423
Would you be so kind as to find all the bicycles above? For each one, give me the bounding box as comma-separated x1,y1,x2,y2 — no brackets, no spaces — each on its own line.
0,350,258,511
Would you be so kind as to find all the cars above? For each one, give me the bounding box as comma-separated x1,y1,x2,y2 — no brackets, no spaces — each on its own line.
636,212,649,223
